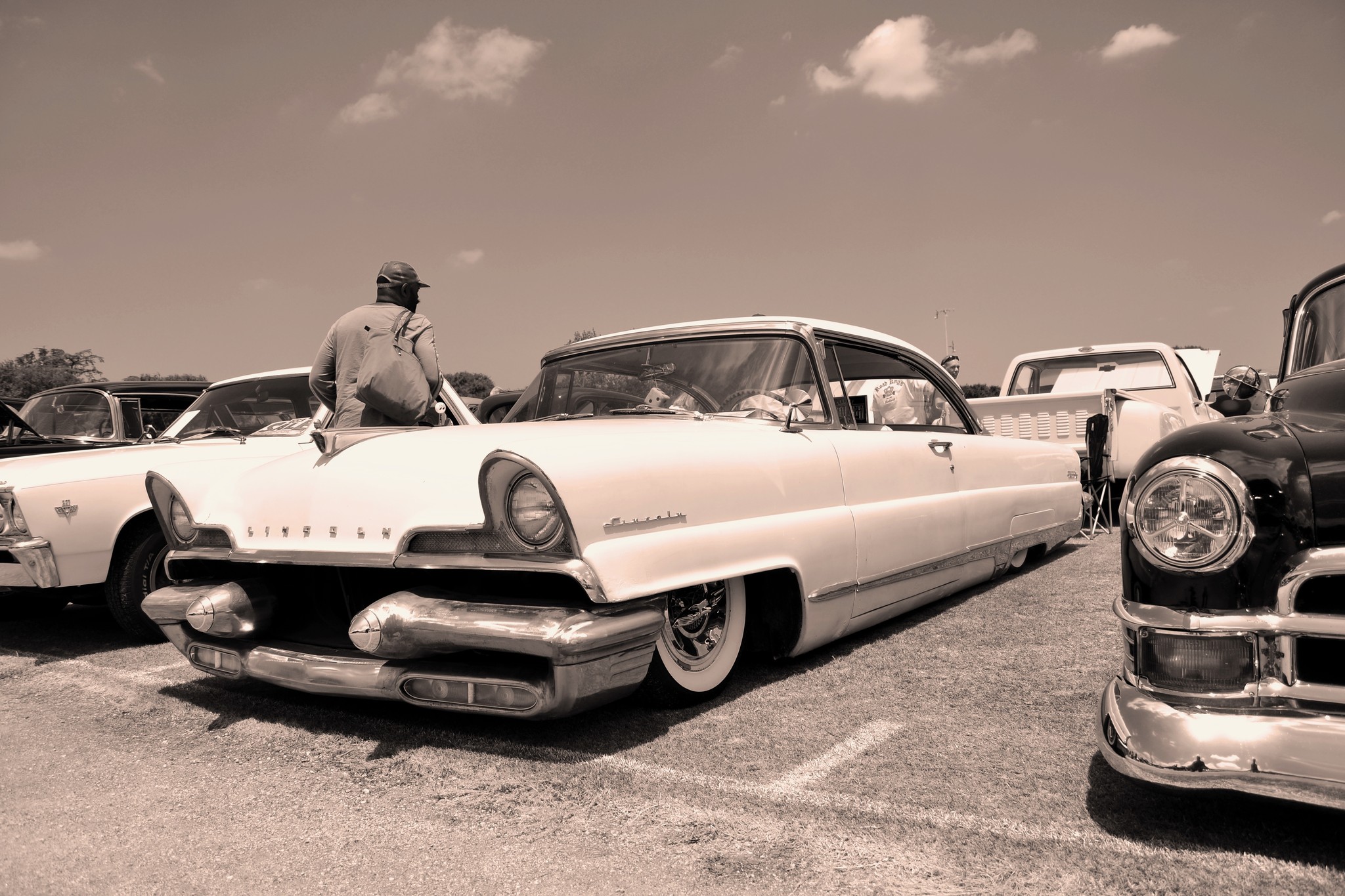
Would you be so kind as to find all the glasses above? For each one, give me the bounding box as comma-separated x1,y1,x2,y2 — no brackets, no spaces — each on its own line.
942,355,960,365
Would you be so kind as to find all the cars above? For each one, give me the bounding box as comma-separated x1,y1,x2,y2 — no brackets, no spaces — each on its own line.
1094,267,1344,816
137,314,1088,722
0,359,523,644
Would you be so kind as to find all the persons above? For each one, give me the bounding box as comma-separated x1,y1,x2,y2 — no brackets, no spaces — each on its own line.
871,379,932,427
485,386,517,424
924,356,961,430
669,392,701,412
309,261,443,428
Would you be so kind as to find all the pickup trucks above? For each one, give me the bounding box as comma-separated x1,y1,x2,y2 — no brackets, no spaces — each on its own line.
940,341,1227,507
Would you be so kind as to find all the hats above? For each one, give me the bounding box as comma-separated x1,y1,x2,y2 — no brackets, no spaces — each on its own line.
376,261,431,289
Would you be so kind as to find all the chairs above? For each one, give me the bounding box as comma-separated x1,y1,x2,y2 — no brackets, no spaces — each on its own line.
1075,412,1111,541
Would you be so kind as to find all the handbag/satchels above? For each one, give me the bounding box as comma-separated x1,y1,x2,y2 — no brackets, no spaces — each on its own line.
355,326,430,423
